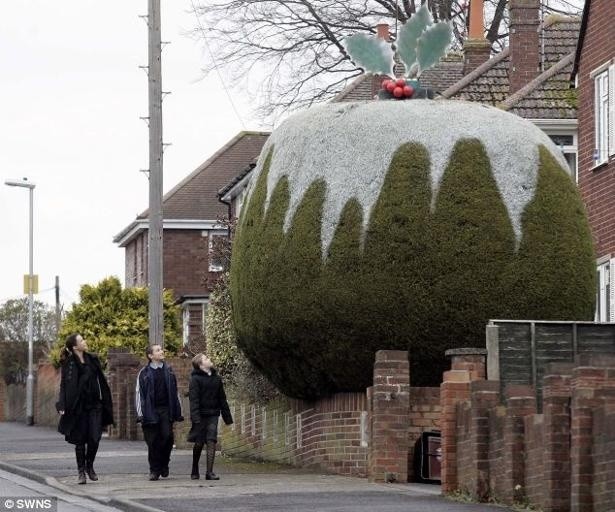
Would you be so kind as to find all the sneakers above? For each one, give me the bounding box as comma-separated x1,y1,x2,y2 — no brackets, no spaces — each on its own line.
78,473,86,485
87,466,98,481
148,467,169,480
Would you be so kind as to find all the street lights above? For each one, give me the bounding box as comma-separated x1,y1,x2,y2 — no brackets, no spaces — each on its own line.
5,177,36,425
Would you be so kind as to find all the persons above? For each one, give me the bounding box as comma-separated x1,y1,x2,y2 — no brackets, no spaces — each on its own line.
56,332,116,484
186,352,234,480
134,344,185,479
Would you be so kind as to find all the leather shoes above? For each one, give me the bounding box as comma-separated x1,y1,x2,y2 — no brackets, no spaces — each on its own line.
205,470,222,479
189,473,199,479
